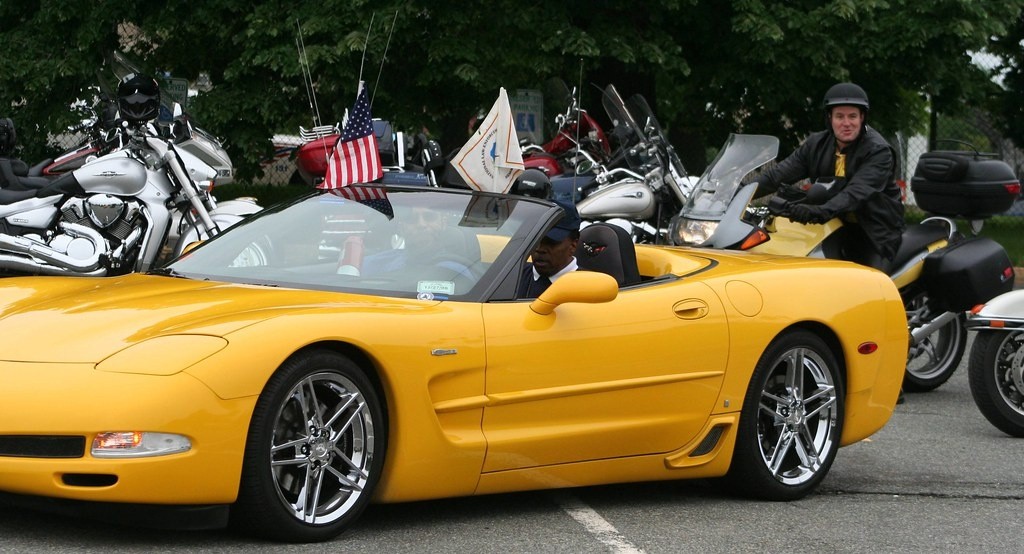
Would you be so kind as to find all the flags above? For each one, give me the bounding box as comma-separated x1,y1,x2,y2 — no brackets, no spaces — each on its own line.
450,87,525,193
458,191,519,231
315,85,384,189
328,187,396,221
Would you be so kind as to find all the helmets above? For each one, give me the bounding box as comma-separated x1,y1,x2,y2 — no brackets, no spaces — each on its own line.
115,72,161,125
823,83,870,112
511,169,552,199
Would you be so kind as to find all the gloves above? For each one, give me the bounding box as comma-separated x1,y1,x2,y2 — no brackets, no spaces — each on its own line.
789,204,823,226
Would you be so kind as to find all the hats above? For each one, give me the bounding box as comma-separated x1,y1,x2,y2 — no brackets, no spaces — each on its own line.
544,198,580,243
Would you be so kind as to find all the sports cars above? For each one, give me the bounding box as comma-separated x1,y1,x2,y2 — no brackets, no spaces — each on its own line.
0,184,915,544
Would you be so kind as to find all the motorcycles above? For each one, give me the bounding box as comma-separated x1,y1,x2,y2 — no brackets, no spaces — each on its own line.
290,81,1024,442
0,48,280,288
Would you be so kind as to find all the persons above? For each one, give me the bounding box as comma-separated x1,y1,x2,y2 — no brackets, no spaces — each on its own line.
342,198,483,298
728,83,907,403
518,199,602,301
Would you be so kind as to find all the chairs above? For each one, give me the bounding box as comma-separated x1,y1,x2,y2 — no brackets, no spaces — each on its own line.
440,226,482,263
575,223,640,287
